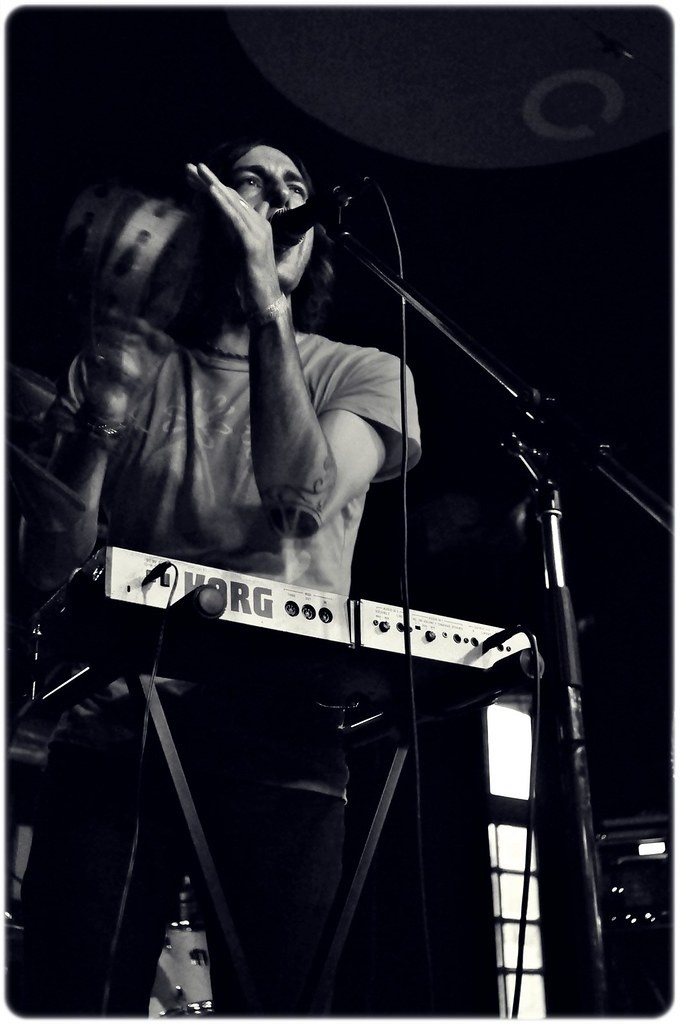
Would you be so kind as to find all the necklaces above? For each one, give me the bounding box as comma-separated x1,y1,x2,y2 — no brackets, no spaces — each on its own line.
190,337,248,360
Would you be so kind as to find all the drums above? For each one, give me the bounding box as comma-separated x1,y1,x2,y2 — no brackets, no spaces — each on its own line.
99,192,194,319
149,920,214,1014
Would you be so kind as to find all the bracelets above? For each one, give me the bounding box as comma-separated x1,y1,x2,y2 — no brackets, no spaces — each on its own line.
73,408,126,445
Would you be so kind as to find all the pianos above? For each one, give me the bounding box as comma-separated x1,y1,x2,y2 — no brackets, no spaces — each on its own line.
27,540,550,753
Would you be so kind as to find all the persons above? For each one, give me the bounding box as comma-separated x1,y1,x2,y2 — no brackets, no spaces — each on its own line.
6,134,421,1017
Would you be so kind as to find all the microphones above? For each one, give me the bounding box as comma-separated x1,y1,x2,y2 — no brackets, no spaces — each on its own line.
267,176,376,249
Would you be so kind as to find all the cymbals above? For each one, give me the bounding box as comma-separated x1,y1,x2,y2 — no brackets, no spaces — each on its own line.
109,244,138,283
151,198,174,218
